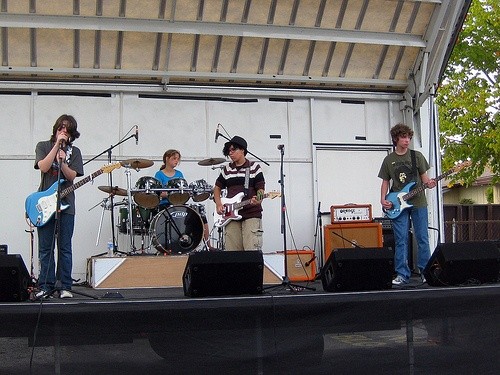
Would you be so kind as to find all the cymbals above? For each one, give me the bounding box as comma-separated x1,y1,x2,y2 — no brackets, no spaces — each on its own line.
98,186,127,196
198,158,226,166
121,158,154,168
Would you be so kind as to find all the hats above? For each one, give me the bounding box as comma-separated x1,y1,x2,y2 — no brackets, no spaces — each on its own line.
225,136,248,156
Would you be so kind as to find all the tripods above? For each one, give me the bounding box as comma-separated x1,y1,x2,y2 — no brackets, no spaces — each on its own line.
82,134,136,257
32,139,99,300
263,145,316,294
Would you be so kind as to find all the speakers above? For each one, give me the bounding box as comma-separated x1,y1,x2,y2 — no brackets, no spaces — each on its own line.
382,229,414,271
0,254,31,302
320,247,394,292
284,249,316,281
423,240,500,287
182,250,264,298
323,223,383,262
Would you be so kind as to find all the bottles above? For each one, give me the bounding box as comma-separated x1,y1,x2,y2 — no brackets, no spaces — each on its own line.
107,237,113,256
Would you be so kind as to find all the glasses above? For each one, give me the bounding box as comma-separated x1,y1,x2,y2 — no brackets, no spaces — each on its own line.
228,148,236,153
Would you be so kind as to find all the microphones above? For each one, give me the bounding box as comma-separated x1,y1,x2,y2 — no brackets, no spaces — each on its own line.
305,256,316,266
215,125,219,143
277,145,284,150
136,126,139,145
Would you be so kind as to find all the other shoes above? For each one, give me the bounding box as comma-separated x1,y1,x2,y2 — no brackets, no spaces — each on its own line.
36,290,53,299
421,273,426,282
59,290,73,298
392,276,402,284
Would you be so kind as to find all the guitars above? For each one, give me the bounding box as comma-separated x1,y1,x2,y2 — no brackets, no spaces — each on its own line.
25,162,121,227
383,161,473,219
214,192,282,227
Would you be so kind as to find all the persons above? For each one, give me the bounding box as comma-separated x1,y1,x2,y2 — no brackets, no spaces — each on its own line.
34,114,83,299
155,149,214,251
378,124,437,285
214,136,265,250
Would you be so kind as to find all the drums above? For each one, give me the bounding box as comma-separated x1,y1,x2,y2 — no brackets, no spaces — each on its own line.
117,205,154,235
188,179,210,202
168,177,190,205
149,205,205,255
190,204,205,217
134,175,161,209
